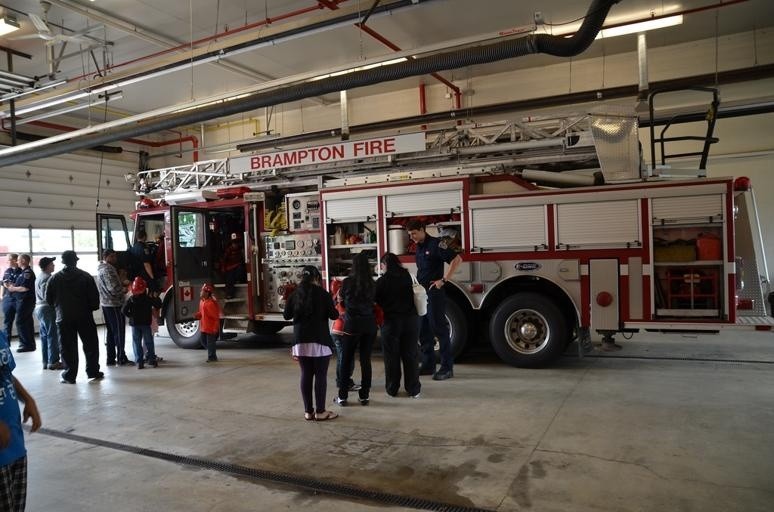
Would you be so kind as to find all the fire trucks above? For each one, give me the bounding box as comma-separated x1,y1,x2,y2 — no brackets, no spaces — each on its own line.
93,86,773,372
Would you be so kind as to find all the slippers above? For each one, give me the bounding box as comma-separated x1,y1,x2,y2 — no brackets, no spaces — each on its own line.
316,411,338,420
304,412,315,420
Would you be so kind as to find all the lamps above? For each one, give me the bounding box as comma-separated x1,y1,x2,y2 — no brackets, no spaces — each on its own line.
563,14,687,40
0,14,20,40
2,88,126,130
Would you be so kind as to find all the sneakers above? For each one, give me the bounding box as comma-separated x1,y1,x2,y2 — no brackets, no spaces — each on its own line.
87,372,105,379
359,397,369,405
418,366,436,376
107,357,163,369
42,363,62,370
432,370,454,380
348,384,361,390
333,397,345,406
62,372,76,384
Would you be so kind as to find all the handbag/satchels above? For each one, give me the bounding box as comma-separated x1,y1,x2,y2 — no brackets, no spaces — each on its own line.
410,271,428,316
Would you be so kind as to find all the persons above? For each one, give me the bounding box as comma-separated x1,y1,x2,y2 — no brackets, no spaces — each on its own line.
406,220,462,380
194,285,221,361
0,331,41,512
0,231,162,384
331,281,362,391
333,253,377,406
283,265,339,420
376,253,422,399
221,233,242,300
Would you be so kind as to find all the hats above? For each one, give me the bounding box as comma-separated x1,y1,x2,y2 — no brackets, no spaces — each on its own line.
102,249,114,260
39,256,56,268
62,251,79,263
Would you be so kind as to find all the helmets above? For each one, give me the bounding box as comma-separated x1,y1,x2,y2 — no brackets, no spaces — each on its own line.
200,283,213,293
131,276,147,294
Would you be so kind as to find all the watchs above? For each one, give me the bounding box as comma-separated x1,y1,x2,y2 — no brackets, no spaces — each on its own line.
442,277,446,283
